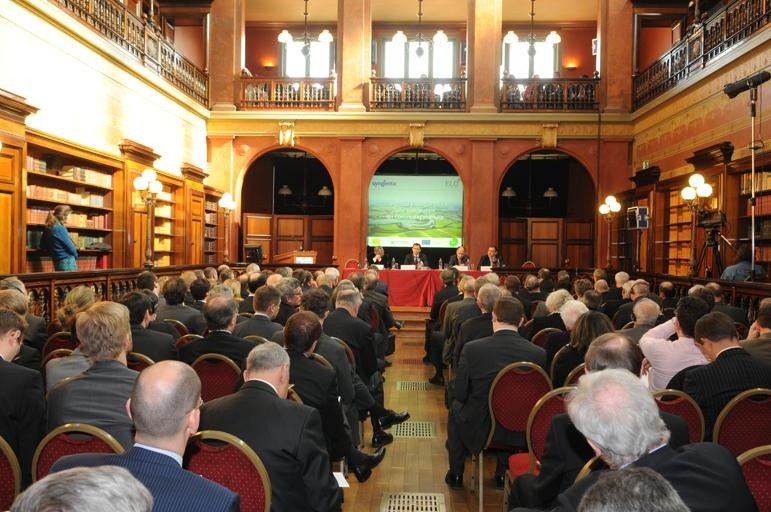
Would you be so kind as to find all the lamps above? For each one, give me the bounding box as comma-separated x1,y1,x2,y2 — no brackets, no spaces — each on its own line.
597,195,622,225
544,28,562,44
431,30,448,43
543,187,557,198
318,185,331,196
218,190,237,217
391,29,408,43
276,28,292,43
681,173,713,216
318,27,334,42
261,59,274,73
501,187,515,197
133,167,163,202
277,184,292,195
563,62,577,72
502,29,520,45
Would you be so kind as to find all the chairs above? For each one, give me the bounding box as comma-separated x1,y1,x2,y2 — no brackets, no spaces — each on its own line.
652,388,705,446
563,362,586,399
0,437,22,512
174,335,203,355
736,445,771,512
503,386,577,512
31,422,127,483
191,353,244,403
468,362,552,512
42,332,78,362
713,388,771,456
180,430,271,512
161,317,188,340
528,326,562,350
125,351,154,373
40,349,71,374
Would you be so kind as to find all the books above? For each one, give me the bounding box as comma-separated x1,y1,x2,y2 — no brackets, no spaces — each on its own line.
745,195,771,215
68,212,108,230
100,255,107,269
67,231,104,251
20,153,113,186
25,228,48,247
25,183,104,207
26,204,55,225
25,255,56,273
739,170,771,194
203,201,218,265
77,256,97,269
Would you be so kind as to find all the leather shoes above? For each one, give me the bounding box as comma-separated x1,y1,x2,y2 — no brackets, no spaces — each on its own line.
429,376,444,385
354,446,385,484
394,319,404,328
372,432,393,447
445,470,462,490
496,474,505,490
380,411,410,430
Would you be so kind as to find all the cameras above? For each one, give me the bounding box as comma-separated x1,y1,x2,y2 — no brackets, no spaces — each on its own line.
695,210,728,226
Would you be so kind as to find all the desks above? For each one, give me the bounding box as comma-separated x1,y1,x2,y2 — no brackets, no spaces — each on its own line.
341,268,492,307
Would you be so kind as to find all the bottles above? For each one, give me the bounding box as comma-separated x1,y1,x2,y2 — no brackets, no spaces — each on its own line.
391,257,396,269
438,258,443,269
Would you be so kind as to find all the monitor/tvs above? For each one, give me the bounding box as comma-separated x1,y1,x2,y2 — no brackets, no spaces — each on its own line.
243,243,264,263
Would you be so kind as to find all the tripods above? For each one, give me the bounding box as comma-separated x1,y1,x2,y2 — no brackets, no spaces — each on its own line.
692,226,727,279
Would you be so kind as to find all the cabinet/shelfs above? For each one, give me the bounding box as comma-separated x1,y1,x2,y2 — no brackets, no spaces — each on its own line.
613,140,767,282
0,87,225,273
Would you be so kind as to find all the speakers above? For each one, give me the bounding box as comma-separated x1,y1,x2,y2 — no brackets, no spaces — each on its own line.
628,205,648,229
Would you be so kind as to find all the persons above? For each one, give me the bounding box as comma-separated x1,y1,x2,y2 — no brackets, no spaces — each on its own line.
281,310,386,484
574,279,593,302
47,285,98,336
267,274,281,287
326,267,339,288
362,272,394,351
300,288,331,323
276,278,305,323
477,243,505,272
524,274,539,292
639,297,712,401
49,360,241,512
237,272,266,313
689,285,705,296
594,279,610,304
301,289,410,446
613,279,656,329
422,270,458,364
606,280,634,318
504,333,692,511
692,288,715,312
237,273,249,298
545,300,591,357
204,267,219,282
157,276,171,306
43,204,80,271
220,270,235,281
722,244,763,282
122,290,174,362
569,72,594,102
304,271,318,289
618,298,659,349
443,295,547,490
528,278,554,301
246,263,260,275
556,368,756,511
365,269,405,329
428,276,475,384
682,312,771,444
577,466,690,512
1,288,40,368
180,270,197,304
660,281,679,309
316,273,335,296
291,270,307,291
368,266,388,296
135,271,164,303
504,275,530,318
537,268,549,282
10,465,155,512
738,296,771,367
330,277,392,373
224,279,240,298
142,289,180,338
1,307,44,492
544,71,563,101
582,289,604,312
370,245,390,269
199,342,343,512
324,288,393,447
190,278,210,310
235,287,283,342
532,279,571,320
448,246,470,269
177,294,259,368
405,242,430,270
48,301,139,450
593,268,608,282
524,74,545,108
528,289,574,341
348,271,386,358
217,264,229,282
0,277,44,348
507,74,521,108
705,282,750,327
155,278,207,335
602,280,636,320
453,284,506,372
442,277,494,367
599,271,629,306
242,68,263,101
553,311,618,388
